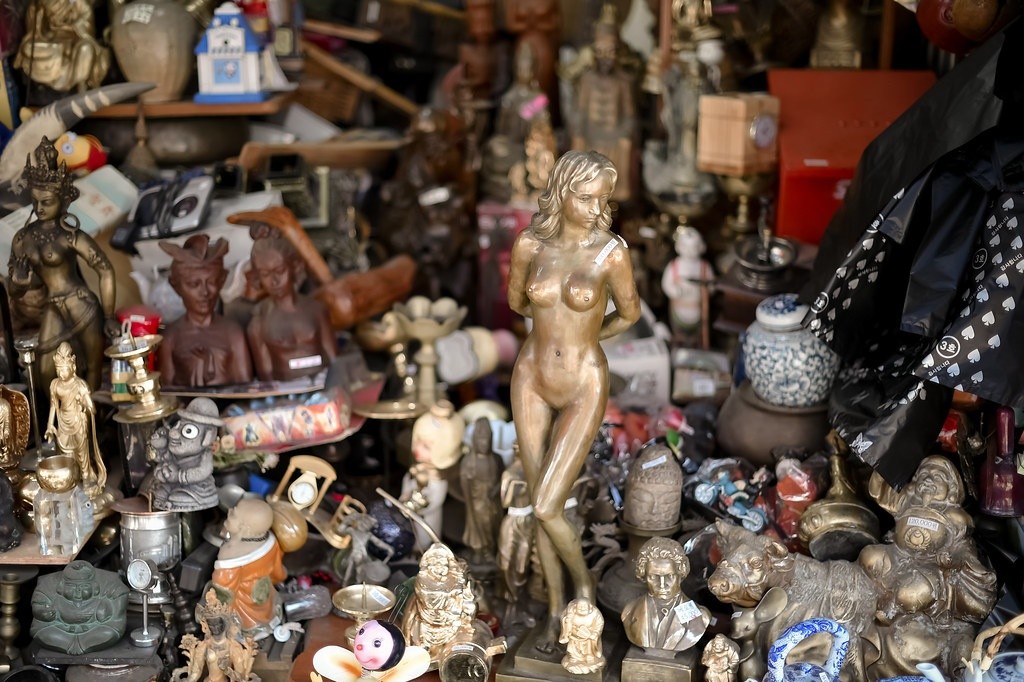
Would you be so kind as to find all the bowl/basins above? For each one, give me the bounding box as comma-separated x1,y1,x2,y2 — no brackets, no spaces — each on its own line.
37,455,81,492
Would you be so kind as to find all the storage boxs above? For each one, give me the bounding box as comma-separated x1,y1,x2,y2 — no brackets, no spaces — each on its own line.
697,93,779,175
768,70,934,241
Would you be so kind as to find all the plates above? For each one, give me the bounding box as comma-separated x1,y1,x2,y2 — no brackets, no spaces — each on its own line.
332,584,397,615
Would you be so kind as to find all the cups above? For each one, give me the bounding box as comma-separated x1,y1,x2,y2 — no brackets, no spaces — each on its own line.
439,618,508,682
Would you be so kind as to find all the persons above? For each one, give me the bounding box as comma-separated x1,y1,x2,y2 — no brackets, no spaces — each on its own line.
0,0,998,682
507,151,642,652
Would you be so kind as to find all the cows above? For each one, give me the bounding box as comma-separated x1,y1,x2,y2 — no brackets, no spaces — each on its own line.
709,517,877,682
